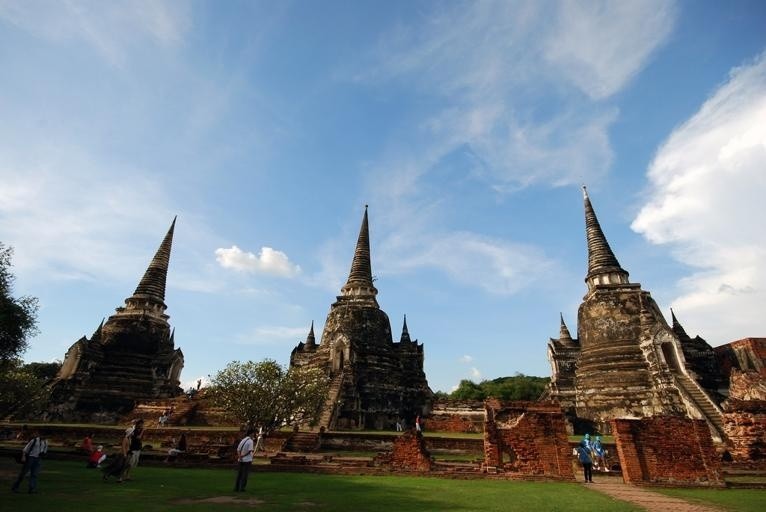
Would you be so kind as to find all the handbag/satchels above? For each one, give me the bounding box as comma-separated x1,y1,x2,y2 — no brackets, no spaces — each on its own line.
15,449,27,464
86,462,98,468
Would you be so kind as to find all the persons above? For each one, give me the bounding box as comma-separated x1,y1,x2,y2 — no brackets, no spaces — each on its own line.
416,415,421,431
584,434,596,470
11,431,48,494
233,429,255,492
80,379,201,483
592,436,609,471
396,415,402,432
577,440,595,483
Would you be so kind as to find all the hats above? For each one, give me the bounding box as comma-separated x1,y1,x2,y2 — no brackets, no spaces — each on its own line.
596,436,600,441
585,434,590,439
125,427,135,438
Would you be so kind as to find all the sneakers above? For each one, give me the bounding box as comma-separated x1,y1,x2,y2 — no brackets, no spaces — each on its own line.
117,478,132,484
593,466,596,470
29,490,39,494
12,488,20,493
598,466,600,471
604,467,610,472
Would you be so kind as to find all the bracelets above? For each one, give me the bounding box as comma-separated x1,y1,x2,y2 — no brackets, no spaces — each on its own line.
22,454,25,456
